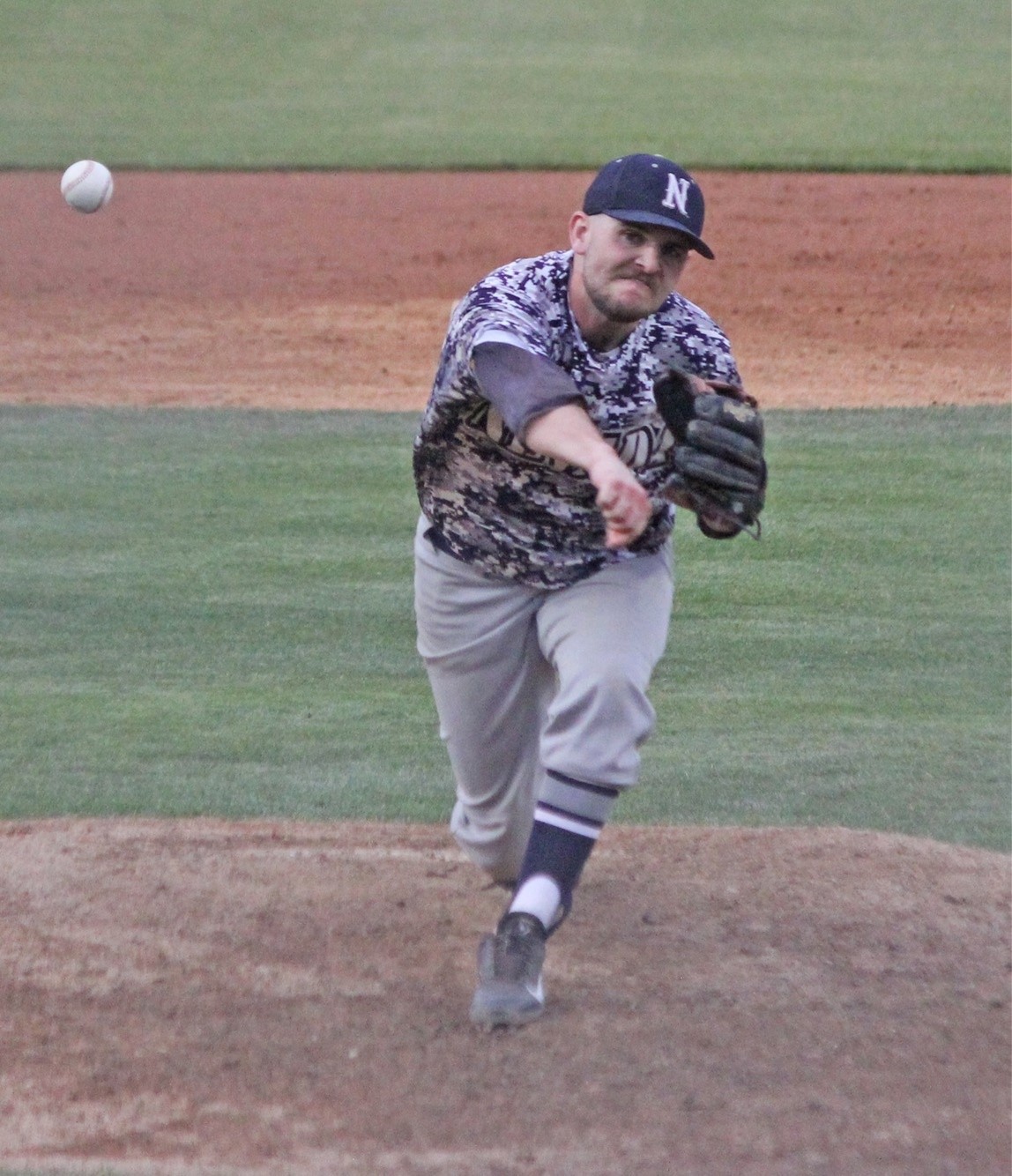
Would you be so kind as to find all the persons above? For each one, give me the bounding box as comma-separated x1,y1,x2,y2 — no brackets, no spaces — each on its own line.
413,153,767,1025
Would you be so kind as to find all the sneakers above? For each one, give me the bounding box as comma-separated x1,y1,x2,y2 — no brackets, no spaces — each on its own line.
473,911,546,1027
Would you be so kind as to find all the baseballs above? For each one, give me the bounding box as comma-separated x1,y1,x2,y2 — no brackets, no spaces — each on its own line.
60,159,113,211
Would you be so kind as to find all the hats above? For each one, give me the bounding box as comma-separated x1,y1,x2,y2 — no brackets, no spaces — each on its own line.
582,154,715,261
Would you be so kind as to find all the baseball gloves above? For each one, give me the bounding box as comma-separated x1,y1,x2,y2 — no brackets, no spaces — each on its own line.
654,367,768,521
